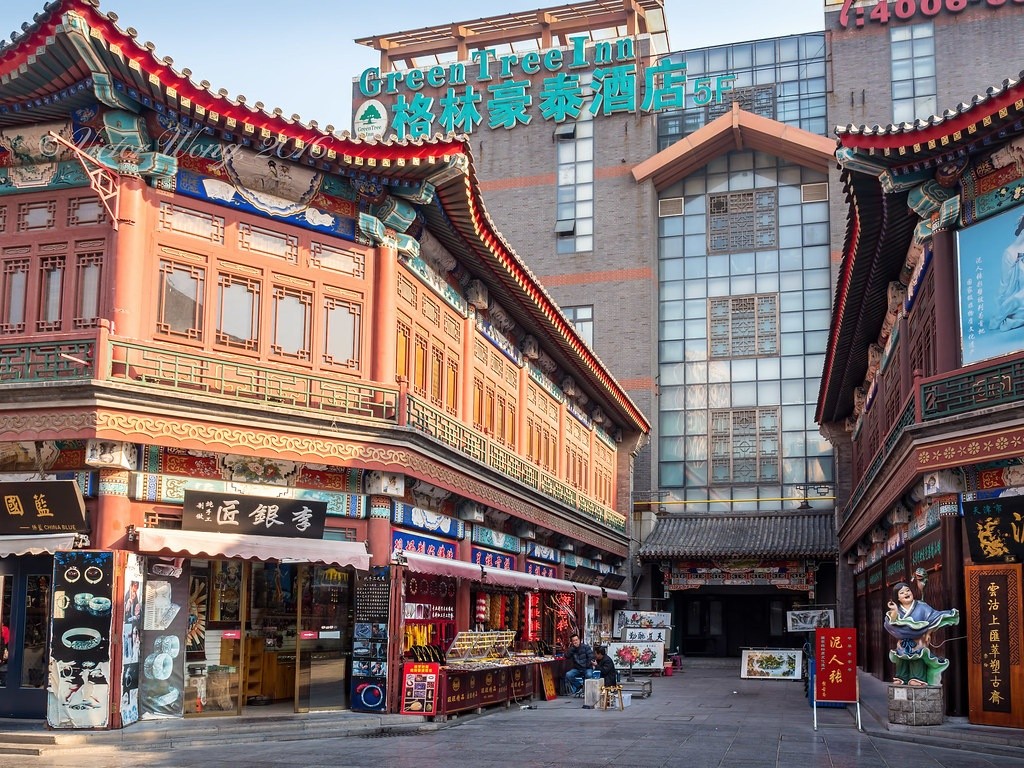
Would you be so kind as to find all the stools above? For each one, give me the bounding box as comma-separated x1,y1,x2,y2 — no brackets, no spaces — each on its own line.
670,655,682,670
603,688,624,711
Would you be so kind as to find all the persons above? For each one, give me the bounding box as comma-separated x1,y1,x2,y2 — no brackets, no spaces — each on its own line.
1000,214,1024,332
0,622,10,664
359,662,382,676
122,581,141,704
221,561,240,621
564,634,594,697
591,646,616,707
884,582,959,685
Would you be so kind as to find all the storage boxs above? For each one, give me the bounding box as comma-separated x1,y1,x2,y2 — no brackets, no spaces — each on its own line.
614,692,632,707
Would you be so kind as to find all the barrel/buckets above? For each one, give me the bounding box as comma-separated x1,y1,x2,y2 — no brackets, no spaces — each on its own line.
665,667,673,676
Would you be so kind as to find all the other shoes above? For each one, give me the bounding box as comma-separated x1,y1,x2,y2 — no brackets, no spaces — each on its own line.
576,688,583,694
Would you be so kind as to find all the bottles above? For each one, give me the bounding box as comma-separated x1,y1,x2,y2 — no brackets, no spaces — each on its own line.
617,670,620,682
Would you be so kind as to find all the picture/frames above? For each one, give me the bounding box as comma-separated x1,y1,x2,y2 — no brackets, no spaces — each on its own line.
606,608,672,670
739,648,803,680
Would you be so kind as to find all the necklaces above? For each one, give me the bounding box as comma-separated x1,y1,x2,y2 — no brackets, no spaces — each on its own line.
227,575,238,587
410,644,446,662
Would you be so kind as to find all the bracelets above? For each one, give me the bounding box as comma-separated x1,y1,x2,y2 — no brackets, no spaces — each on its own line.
410,578,455,597
361,685,383,707
74,592,93,605
89,597,111,611
62,627,101,650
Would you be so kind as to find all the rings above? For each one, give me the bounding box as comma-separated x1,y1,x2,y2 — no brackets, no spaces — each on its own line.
154,686,179,707
57,595,71,609
143,635,180,680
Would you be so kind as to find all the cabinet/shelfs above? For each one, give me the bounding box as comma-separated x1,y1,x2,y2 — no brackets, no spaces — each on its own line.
220,634,264,697
